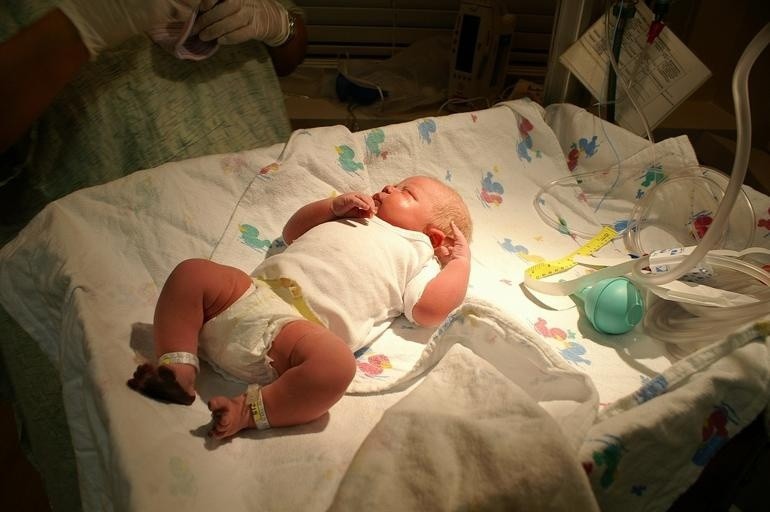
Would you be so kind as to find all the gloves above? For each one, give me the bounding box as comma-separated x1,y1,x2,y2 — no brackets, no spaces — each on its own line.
56,0,291,62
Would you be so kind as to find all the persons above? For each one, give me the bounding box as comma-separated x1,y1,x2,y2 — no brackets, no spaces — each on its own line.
133,176,471,440
0,0,307,229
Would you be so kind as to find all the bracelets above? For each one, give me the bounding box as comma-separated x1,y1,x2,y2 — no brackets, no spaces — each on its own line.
282,12,296,49
